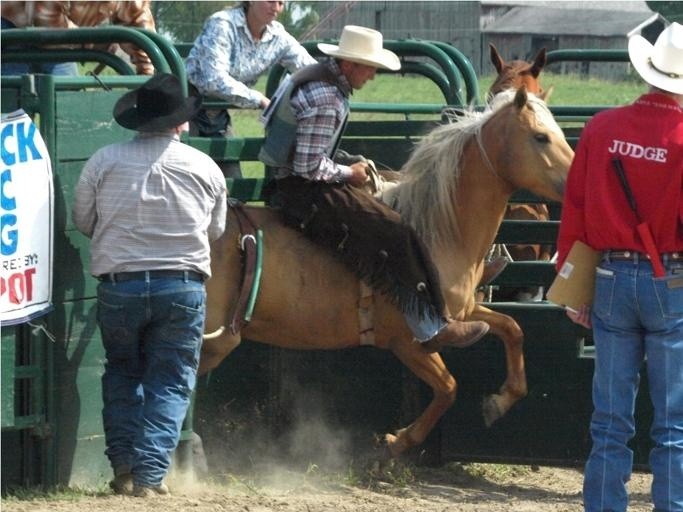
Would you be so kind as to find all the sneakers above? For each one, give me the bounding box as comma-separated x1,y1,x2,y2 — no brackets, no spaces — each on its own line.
106,465,135,498
131,478,171,501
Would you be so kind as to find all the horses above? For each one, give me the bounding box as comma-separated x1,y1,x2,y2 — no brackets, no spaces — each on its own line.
483,43,552,301
186,86,576,477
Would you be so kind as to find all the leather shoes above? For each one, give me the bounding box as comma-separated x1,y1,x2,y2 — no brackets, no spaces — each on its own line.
416,316,491,354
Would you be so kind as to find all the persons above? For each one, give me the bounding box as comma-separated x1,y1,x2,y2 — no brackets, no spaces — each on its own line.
548,17,683,509
181,2,320,139
0,1,168,77
257,23,491,355
70,69,232,497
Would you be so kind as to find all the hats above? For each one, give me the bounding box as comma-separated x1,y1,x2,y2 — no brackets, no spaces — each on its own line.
112,70,204,135
621,12,683,95
316,22,403,73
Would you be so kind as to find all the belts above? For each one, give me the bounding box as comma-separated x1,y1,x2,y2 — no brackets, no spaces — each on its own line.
600,250,682,264
95,266,208,288
